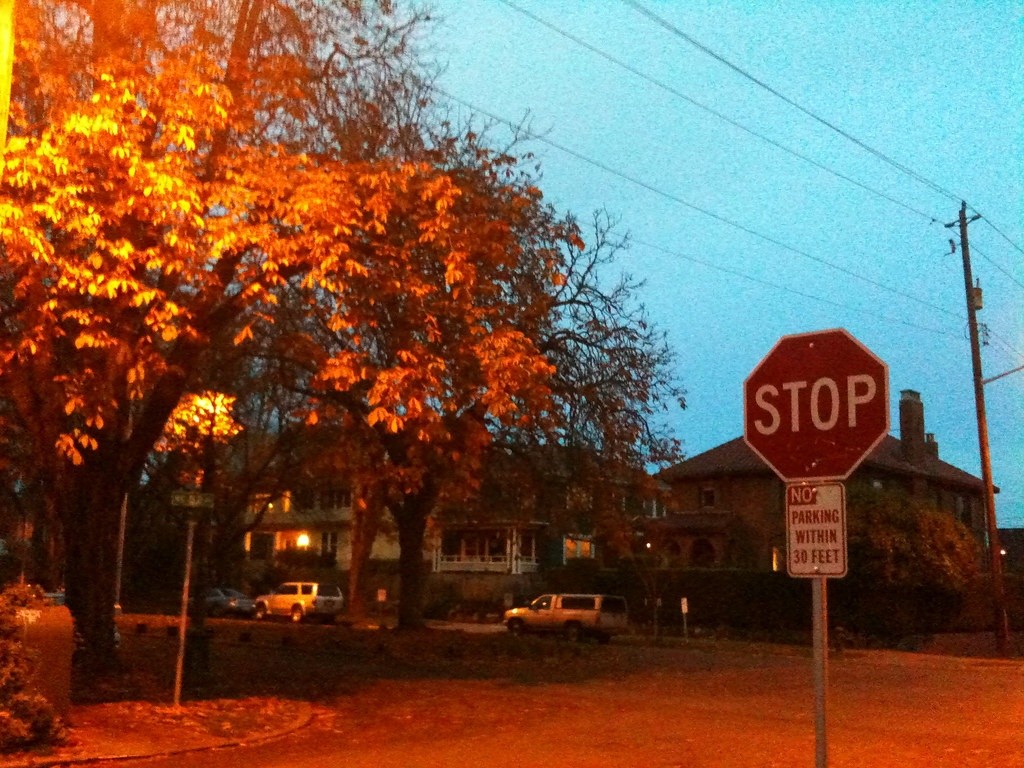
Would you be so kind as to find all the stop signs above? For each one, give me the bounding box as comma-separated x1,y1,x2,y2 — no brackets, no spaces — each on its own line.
741,329,892,488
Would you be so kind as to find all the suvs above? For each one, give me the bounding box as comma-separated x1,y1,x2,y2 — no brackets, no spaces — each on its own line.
256,582,344,624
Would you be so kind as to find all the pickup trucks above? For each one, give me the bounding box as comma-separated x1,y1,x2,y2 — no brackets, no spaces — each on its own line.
505,592,628,639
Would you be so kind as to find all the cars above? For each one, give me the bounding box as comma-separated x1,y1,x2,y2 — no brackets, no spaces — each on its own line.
188,588,257,618
